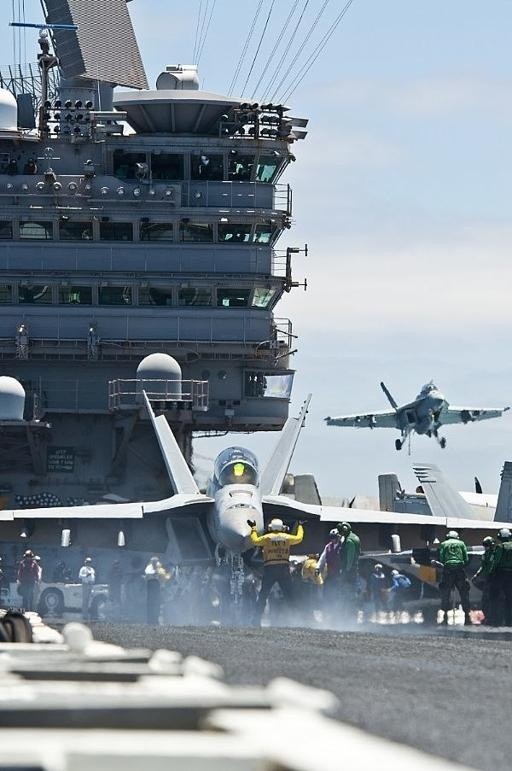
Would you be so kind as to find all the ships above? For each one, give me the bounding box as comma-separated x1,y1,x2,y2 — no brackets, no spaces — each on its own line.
0,0,512,770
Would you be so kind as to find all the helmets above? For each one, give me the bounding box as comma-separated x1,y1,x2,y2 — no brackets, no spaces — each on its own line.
390,568,399,578
497,527,511,539
84,556,93,564
267,518,290,534
22,548,34,558
36,556,42,562
445,530,461,540
482,534,496,546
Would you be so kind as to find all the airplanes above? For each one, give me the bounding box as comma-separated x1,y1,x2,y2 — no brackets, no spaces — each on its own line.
0,388,512,622
1,389,512,570
377,459,512,522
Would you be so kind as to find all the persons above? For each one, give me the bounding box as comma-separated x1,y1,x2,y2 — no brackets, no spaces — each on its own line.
299,553,324,621
336,522,363,628
144,558,169,624
50,561,65,580
249,375,257,398
64,569,75,582
391,570,414,609
107,560,127,612
30,555,43,612
485,529,512,626
19,550,40,608
435,531,471,627
314,528,343,626
472,536,497,625
246,519,314,627
79,557,96,621
370,564,392,624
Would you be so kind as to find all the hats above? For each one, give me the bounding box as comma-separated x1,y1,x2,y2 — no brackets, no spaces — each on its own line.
328,527,339,536
337,520,352,536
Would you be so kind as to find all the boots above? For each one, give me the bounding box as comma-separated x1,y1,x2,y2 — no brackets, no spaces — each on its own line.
464,614,472,625
441,617,449,625
